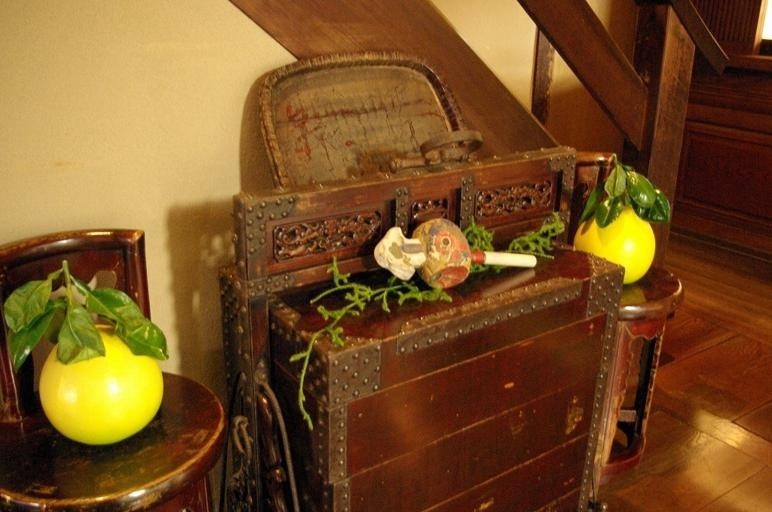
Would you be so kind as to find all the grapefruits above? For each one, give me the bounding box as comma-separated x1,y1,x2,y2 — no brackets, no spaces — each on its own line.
574,153,671,285
4,260,170,446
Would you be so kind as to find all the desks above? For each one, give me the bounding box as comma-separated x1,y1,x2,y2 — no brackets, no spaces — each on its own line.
594,266,684,470
3,370,230,511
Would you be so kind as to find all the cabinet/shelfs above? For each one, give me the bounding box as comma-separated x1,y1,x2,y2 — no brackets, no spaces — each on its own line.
265,238,626,510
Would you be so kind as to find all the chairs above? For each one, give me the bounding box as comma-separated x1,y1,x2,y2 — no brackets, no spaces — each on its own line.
2,226,153,398
